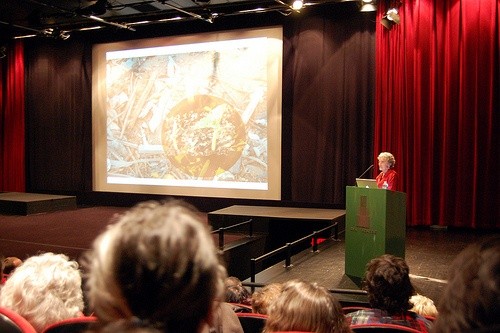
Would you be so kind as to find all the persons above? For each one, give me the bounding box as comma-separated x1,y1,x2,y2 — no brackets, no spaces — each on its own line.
0,251,85,333
80,199,220,333
346,254,432,333
375,151,400,193
432,236,500,333
212,265,345,333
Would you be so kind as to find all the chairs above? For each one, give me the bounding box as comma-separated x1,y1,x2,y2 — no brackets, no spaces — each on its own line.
1,274,436,333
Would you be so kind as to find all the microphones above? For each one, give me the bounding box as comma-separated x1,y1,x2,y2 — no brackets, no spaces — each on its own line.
355,165,374,187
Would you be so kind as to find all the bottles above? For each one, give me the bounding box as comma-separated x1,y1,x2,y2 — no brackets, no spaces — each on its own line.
382,181,388,189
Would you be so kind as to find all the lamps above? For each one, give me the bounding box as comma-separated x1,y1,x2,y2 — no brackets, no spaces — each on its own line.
359,0,377,11
379,8,399,29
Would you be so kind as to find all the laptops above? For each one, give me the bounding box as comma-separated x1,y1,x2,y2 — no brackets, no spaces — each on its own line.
356,178,378,189
